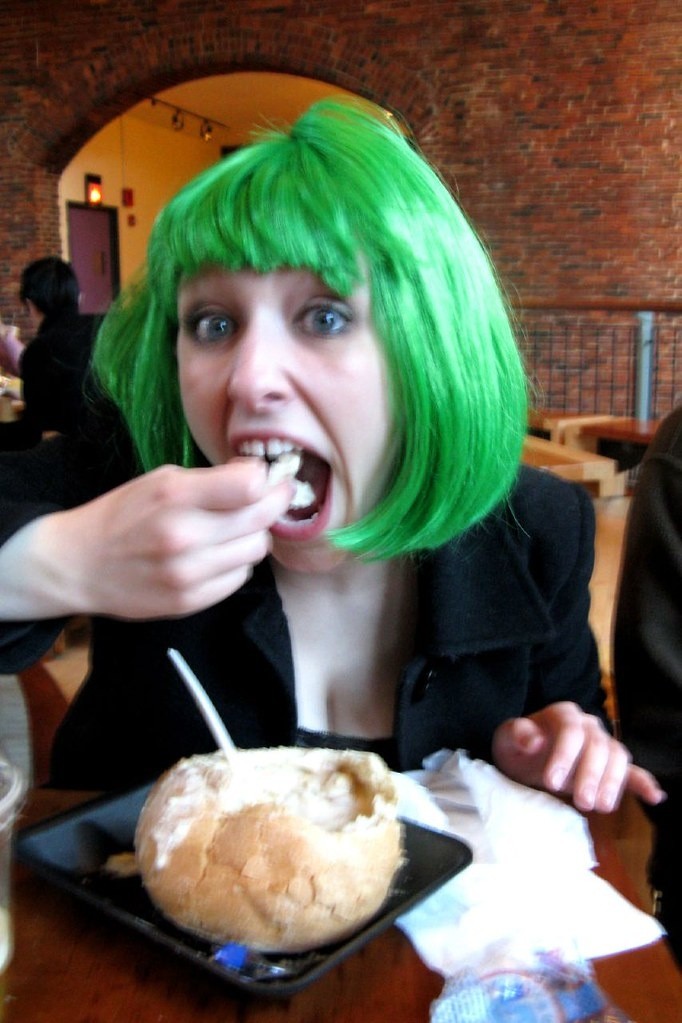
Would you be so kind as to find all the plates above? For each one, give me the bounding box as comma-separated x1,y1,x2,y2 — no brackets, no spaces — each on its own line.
9,775,474,1008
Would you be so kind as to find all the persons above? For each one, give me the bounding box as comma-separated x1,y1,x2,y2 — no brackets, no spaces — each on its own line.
0,256,147,511
0,98,667,816
610,404,682,970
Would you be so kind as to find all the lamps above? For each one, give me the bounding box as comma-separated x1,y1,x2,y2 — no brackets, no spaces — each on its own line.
86,175,103,206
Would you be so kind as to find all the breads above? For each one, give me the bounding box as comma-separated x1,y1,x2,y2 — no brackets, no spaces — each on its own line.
135,742,402,953
261,453,316,510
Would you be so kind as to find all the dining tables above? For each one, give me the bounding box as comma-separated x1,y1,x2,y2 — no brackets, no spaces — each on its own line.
0,786,682,1023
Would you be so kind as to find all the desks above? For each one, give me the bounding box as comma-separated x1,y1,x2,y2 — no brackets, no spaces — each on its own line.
566,419,665,497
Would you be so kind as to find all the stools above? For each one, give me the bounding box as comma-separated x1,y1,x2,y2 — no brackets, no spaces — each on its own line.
521,434,615,501
529,409,609,445
